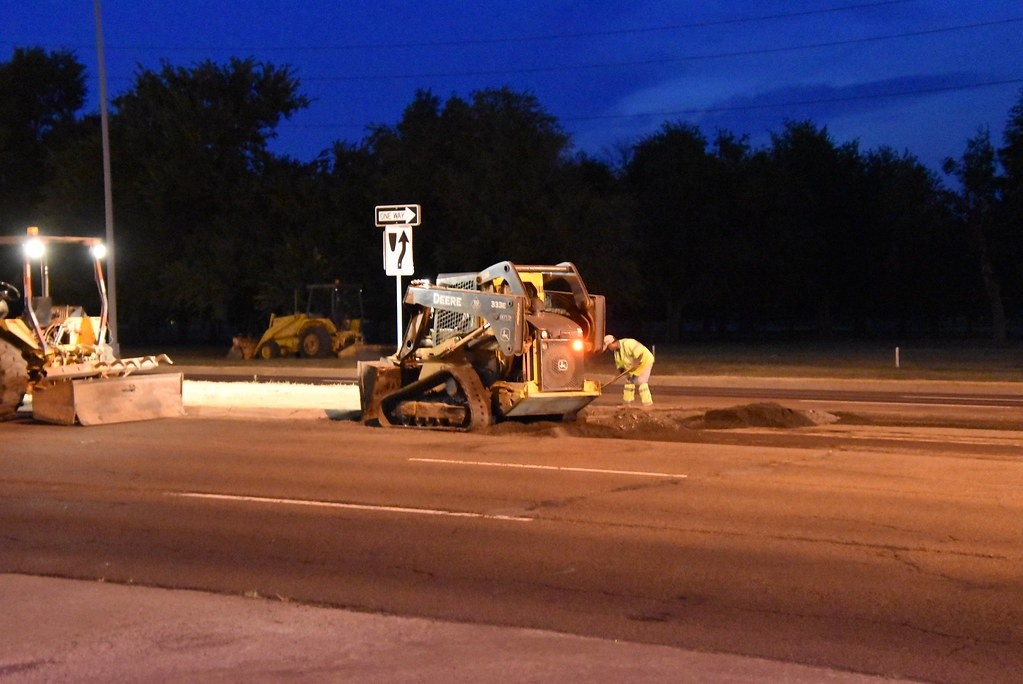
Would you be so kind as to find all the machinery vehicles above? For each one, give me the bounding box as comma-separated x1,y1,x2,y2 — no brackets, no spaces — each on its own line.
1,225,184,426
358,262,606,434
226,279,366,362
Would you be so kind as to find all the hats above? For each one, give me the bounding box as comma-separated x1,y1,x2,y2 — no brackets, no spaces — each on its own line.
603,335,615,350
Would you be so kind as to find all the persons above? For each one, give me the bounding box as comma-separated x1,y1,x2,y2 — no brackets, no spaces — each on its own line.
604,335,655,406
